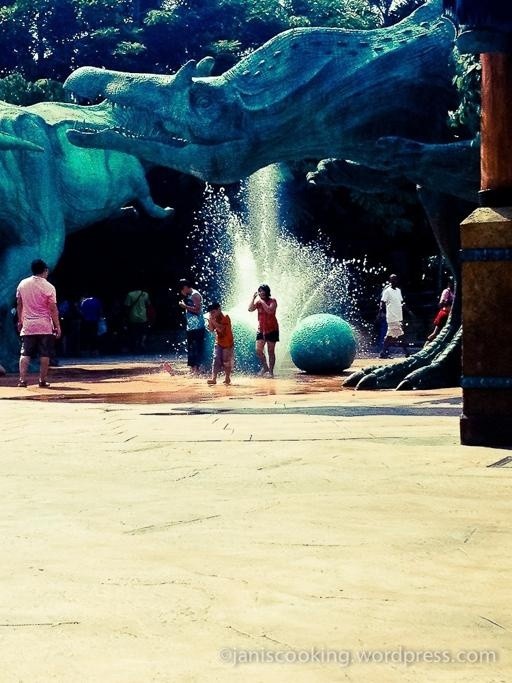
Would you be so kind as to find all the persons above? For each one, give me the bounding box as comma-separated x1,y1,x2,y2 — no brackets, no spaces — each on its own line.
125,285,151,350
249,284,279,378
380,274,411,359
179,278,204,373
58,297,102,357
16,260,61,387
207,303,233,384
428,276,454,341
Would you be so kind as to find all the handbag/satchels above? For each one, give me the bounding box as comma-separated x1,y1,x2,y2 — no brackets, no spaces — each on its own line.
97,318,107,336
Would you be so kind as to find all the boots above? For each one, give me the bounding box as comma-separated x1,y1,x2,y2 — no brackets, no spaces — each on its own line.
18,377,50,387
